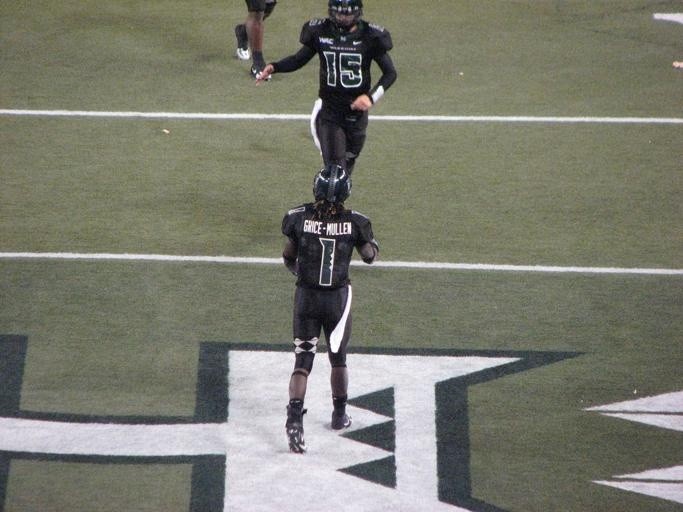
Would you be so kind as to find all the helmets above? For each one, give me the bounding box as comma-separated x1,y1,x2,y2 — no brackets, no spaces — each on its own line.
327,1,363,27
312,164,351,206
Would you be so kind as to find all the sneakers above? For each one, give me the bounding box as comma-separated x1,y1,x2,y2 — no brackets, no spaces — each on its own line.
331,411,351,429
234,24,251,60
285,414,305,453
250,63,271,82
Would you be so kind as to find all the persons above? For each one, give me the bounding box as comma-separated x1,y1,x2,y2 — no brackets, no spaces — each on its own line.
253,0,399,185
278,162,381,457
233,1,276,82
